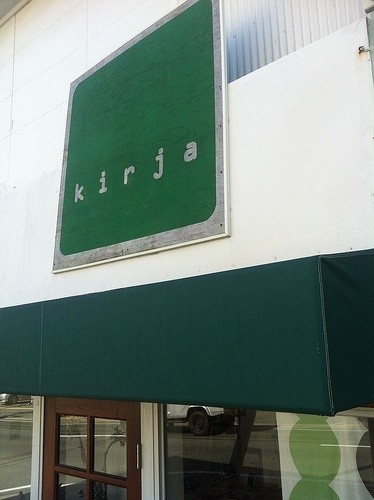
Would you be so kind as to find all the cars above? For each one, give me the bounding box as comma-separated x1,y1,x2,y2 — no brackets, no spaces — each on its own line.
166,403,237,435
0,393,31,405
